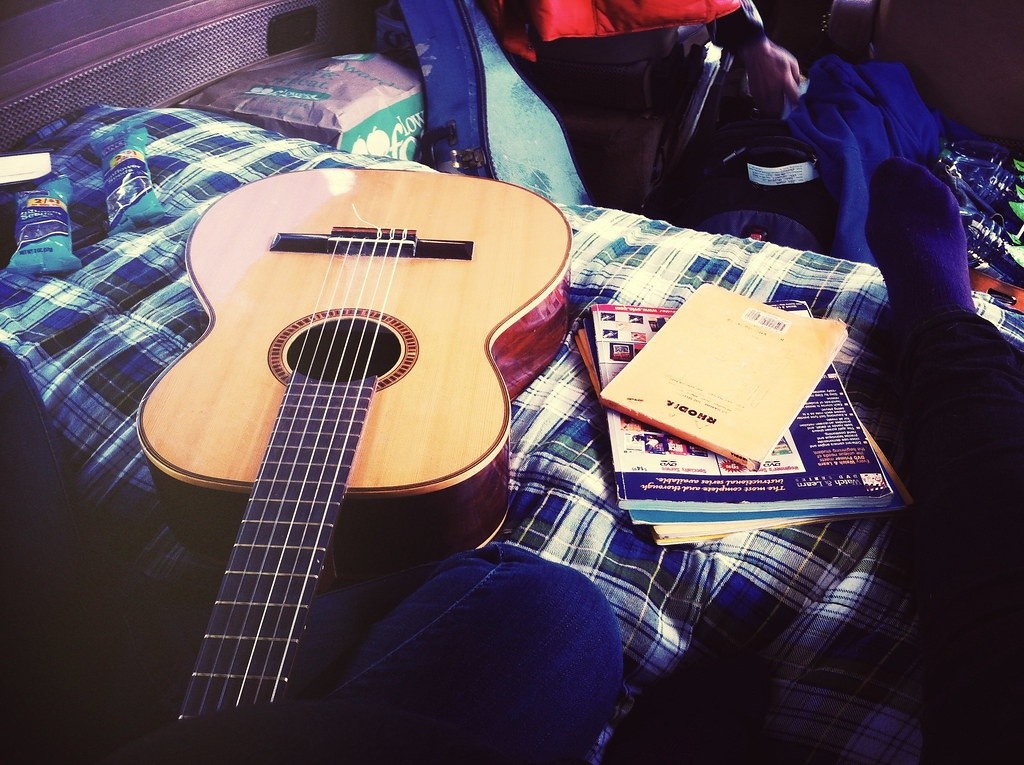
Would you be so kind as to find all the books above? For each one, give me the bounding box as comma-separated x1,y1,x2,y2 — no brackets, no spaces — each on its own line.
573,280,919,546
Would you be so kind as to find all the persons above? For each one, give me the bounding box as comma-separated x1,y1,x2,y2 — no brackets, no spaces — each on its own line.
475,0,803,106
0,343,621,765
609,155,1022,765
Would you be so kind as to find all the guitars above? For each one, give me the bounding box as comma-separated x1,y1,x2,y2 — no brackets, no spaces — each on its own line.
134,165,569,765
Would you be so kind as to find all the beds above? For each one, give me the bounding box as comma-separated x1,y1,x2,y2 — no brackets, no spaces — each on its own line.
0,99,1024,765
